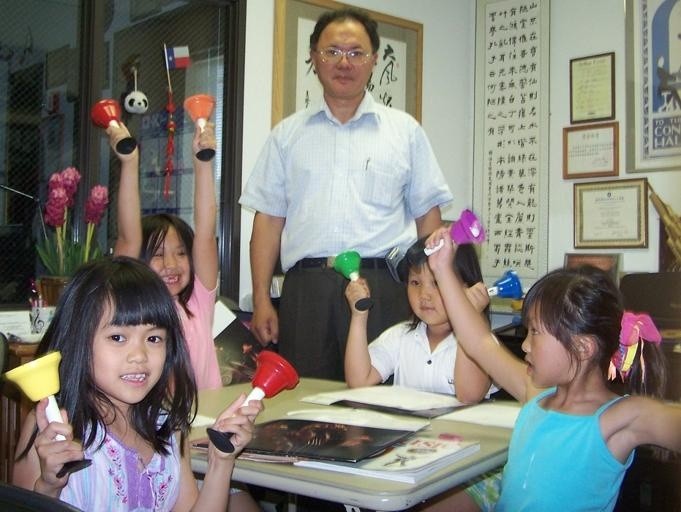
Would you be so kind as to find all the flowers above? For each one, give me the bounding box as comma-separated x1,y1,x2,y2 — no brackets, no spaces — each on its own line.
32,167,108,277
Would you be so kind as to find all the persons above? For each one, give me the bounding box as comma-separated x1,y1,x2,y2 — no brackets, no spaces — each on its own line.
425,227,681,512
345,233,496,406
108,119,223,398
11,249,264,511
237,8,456,380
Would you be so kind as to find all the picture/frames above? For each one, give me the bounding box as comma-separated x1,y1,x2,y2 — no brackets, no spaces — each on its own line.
562,252,622,293
270,0,423,139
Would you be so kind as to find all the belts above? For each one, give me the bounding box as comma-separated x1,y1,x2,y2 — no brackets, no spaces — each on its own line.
295,255,389,271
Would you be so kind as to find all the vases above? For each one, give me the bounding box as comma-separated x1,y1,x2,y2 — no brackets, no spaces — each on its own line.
34,275,69,308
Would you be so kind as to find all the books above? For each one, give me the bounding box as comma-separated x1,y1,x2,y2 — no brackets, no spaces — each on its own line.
191,384,480,483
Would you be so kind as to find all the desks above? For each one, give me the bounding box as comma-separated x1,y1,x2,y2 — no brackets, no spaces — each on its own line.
160,376,523,512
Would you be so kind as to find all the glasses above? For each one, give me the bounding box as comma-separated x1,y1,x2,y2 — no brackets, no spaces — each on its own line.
312,42,375,66
